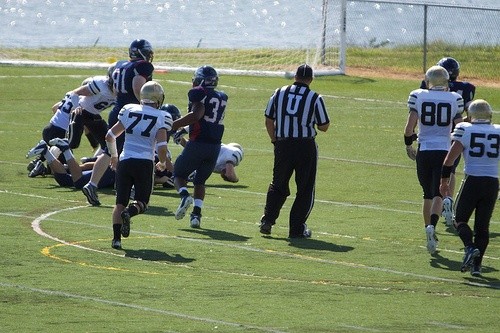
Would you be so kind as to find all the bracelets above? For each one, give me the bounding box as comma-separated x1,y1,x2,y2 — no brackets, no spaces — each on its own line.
404,134,413,145
442,165,452,179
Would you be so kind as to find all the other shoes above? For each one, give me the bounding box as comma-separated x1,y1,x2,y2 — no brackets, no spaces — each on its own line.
426,225,438,254
443,199,453,225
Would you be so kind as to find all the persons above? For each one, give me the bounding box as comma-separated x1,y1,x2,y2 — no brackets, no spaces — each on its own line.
173,65,228,227
403,66,464,253
420,57,476,226
25,40,242,206
259,64,330,238
105,81,172,249
440,99,500,275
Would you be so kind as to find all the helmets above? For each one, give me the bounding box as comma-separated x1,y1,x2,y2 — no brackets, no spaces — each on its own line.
468,99,492,122
191,66,219,88
129,39,154,63
438,57,459,81
227,143,243,160
107,62,117,83
140,81,165,107
161,103,181,119
425,66,450,90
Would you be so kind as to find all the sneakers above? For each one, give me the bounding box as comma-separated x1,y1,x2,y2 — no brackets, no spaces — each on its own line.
460,244,478,273
120,211,130,238
49,138,70,151
289,228,312,238
259,223,271,234
190,214,201,227
29,160,43,177
175,196,193,220
26,139,47,159
111,240,121,248
83,183,101,206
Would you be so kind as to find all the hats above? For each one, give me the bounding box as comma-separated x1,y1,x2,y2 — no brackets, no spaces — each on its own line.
296,65,312,79
